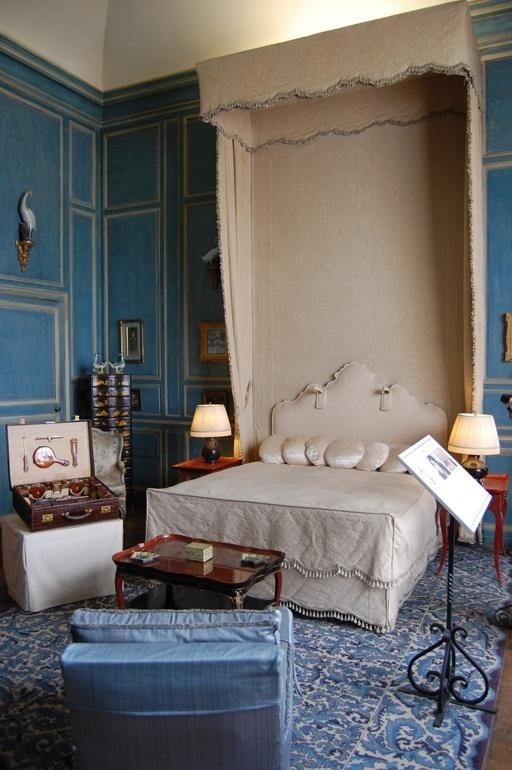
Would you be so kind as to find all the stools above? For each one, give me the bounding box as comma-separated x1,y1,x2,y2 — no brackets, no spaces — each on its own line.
0,513,123,613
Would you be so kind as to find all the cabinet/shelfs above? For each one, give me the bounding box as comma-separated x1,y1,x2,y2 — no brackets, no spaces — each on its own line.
76,372,133,491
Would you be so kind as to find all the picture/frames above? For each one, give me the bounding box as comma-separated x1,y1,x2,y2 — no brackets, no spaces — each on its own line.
199,322,228,363
130,389,142,412
202,391,229,416
119,319,144,363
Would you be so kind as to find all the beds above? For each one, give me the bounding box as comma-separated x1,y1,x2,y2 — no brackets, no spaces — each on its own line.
145,361,446,633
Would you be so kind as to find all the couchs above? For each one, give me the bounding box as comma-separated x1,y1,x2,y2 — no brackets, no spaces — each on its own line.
61,606,295,770
91,428,126,520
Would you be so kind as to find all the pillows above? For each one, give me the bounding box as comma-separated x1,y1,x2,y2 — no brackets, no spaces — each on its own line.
324,437,364,468
282,435,311,466
379,447,409,474
355,442,389,471
305,435,335,467
259,434,286,464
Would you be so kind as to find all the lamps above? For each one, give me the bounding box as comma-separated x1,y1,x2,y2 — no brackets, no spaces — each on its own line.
16,190,37,273
190,402,232,464
202,237,220,292
448,412,500,487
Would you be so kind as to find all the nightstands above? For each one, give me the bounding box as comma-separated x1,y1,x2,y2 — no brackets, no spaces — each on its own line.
170,457,242,480
436,474,509,587
